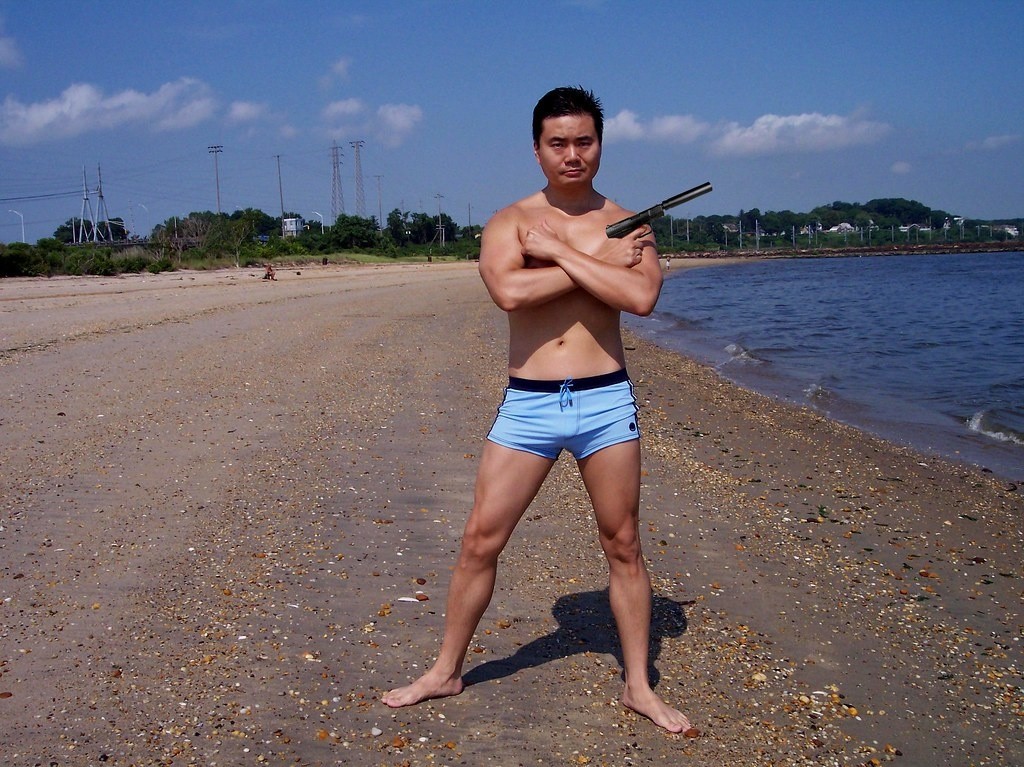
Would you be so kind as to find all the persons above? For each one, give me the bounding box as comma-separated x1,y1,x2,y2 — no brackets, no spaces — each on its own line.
667,257,670,270
381,86,691,734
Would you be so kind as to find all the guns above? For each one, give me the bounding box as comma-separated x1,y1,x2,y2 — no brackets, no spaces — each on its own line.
604,181,712,241
